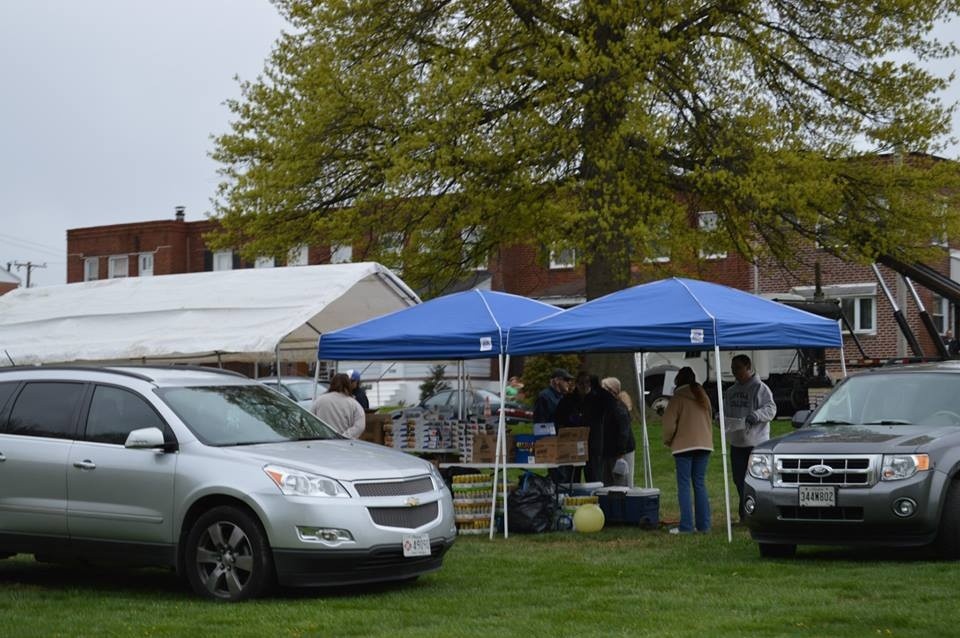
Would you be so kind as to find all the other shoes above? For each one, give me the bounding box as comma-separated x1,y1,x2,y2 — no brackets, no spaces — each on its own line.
670,528,693,535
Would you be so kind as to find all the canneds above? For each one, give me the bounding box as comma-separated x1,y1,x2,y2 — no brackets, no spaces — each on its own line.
559,497,598,525
452,473,515,530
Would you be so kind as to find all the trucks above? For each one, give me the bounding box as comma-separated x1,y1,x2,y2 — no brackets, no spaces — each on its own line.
636,228,959,416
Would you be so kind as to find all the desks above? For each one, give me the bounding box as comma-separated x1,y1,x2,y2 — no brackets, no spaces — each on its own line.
437,461,587,505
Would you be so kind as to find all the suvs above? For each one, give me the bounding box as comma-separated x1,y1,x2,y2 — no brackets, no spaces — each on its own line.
1,365,458,605
740,359,960,559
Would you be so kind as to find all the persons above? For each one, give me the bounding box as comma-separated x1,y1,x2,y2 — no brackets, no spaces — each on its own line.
348,370,369,409
943,330,960,360
310,372,366,440
715,355,777,526
532,370,636,488
664,367,714,535
505,375,525,403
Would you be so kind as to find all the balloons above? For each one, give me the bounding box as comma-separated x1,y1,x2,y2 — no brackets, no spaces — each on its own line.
573,504,604,532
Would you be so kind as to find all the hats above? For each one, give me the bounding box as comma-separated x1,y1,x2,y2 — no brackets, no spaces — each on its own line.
552,368,576,379
604,377,620,393
346,369,360,380
675,367,694,386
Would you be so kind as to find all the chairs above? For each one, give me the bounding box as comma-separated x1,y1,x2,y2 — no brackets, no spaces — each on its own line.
96,395,153,440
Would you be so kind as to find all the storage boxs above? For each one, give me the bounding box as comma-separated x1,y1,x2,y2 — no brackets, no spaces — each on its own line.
359,409,662,535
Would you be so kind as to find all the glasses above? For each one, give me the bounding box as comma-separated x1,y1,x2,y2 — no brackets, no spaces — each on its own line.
556,377,570,382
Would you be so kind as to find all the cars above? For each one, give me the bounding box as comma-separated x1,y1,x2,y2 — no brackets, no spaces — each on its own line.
256,377,327,416
388,386,533,425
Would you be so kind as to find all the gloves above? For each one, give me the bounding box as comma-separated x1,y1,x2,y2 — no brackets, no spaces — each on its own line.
745,416,755,428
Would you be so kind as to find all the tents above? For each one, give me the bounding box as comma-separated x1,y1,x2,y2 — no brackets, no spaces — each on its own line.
489,277,851,544
0,262,427,406
313,290,653,538
